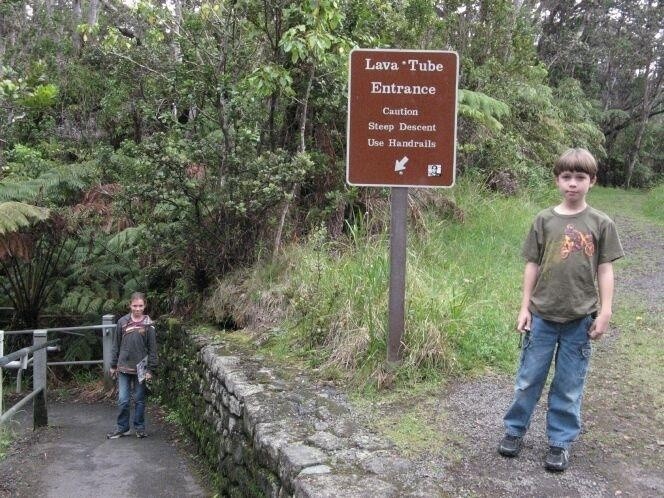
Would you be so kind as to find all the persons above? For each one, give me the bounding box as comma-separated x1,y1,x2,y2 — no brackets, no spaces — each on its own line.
497,147,626,470
105,292,157,440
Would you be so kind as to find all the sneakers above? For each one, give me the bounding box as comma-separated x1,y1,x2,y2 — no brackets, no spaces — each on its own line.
545,444,568,471
106,428,131,439
136,430,148,439
499,430,523,457
